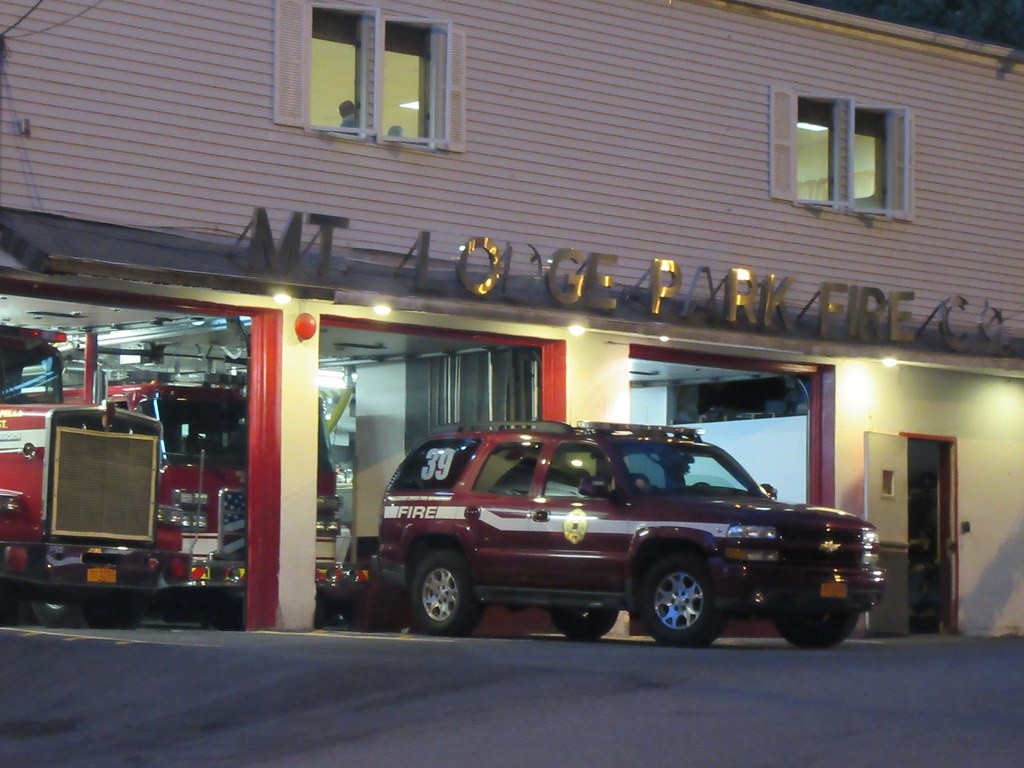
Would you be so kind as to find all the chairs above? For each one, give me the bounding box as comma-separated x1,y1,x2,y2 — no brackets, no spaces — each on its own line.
630,473,652,494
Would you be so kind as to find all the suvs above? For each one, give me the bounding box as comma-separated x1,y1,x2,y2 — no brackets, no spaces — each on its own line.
370,415,887,651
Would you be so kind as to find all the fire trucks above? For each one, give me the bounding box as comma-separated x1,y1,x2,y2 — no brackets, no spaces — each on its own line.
2,313,369,632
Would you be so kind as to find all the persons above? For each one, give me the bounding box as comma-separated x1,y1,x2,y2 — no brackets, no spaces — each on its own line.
339,101,355,128
